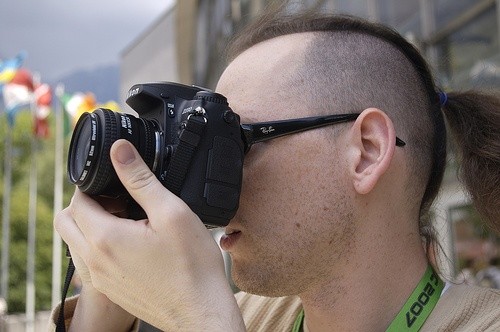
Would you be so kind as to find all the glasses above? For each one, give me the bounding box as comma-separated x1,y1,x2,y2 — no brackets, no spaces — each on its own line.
239,112,406,154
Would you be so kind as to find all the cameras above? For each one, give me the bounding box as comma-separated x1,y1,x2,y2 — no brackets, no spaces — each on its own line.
67,80,243,229
